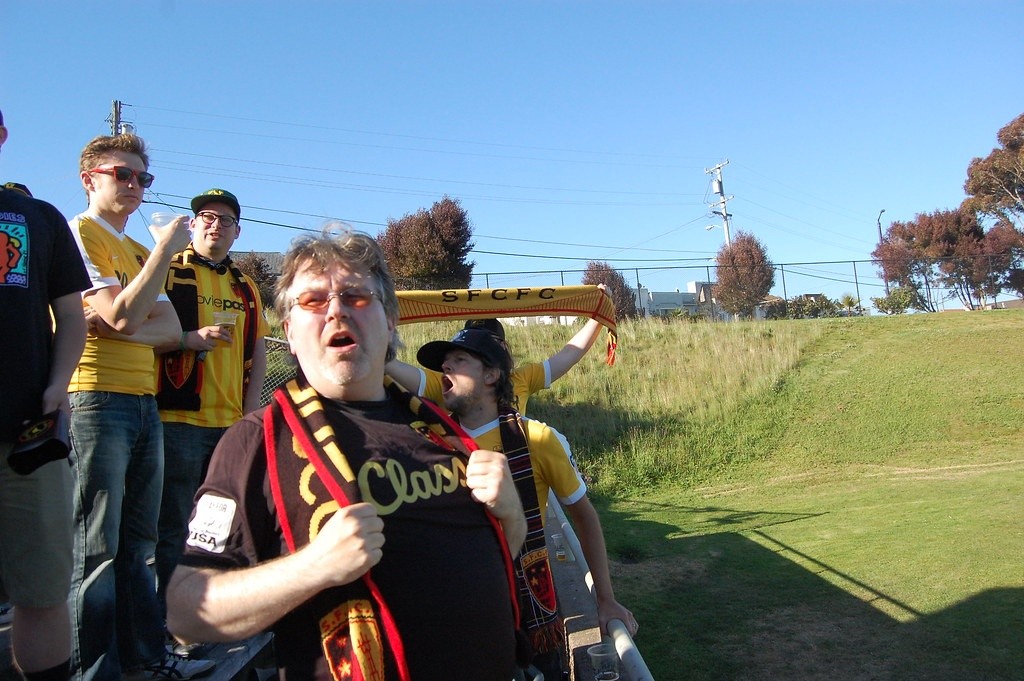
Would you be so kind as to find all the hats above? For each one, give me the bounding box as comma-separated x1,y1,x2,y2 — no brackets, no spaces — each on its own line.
190,188,241,222
0,182,34,197
463,319,506,340
416,329,506,362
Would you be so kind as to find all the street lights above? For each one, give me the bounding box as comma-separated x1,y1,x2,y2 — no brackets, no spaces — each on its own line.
706,224,741,322
876,208,890,296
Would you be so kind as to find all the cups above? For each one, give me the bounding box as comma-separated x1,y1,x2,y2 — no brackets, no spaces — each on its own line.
551,534,566,560
151,212,183,227
587,644,619,681
213,312,240,341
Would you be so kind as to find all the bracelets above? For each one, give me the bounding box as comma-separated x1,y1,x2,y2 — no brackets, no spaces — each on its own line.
179,331,187,352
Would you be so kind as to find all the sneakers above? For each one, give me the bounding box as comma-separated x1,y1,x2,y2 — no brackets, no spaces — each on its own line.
117,652,215,681
162,617,205,657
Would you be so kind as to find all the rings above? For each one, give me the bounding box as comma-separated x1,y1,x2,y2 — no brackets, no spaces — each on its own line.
632,623,638,628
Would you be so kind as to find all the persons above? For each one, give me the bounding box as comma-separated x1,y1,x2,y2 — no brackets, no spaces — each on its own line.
0,112,191,681
159,188,271,657
416,329,638,681
165,222,527,681
384,283,613,417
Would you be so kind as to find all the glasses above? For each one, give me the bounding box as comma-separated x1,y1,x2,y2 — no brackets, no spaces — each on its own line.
289,287,382,311
88,165,155,188
194,211,239,227
